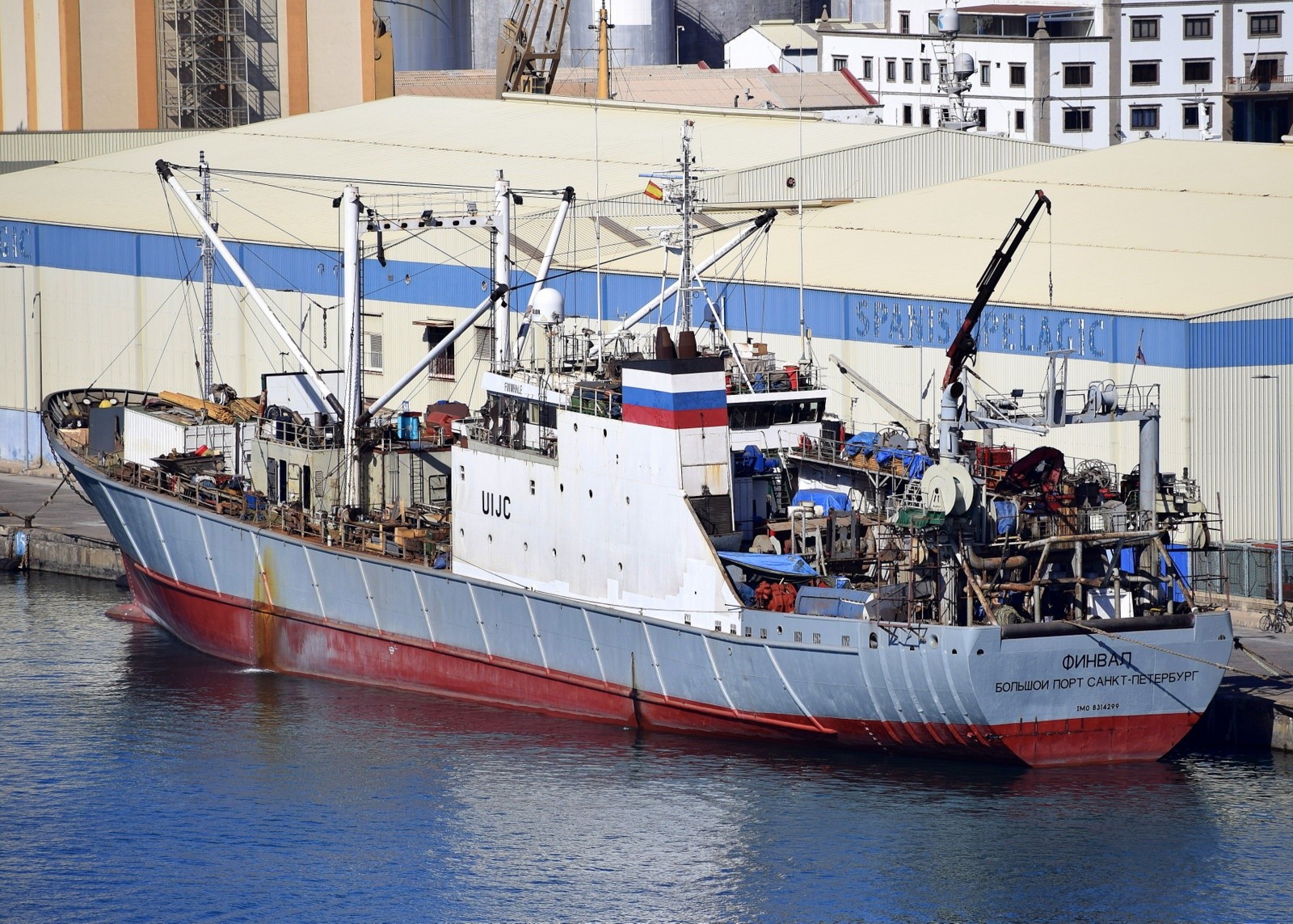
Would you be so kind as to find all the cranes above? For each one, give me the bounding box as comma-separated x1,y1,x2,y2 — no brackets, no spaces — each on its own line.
494,0,621,100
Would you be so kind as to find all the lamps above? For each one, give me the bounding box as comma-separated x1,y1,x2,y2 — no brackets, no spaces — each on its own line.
1174,495,1184,505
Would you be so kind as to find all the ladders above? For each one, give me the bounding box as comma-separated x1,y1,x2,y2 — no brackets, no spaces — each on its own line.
410,453,424,505
771,472,792,509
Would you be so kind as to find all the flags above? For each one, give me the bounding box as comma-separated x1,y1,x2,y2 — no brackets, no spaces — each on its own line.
643,181,663,201
1137,345,1146,363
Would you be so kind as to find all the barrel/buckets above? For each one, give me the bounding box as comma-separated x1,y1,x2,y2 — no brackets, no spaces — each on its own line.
398,414,419,440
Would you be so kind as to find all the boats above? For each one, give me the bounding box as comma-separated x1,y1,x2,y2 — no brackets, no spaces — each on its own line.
40,114,1238,767
